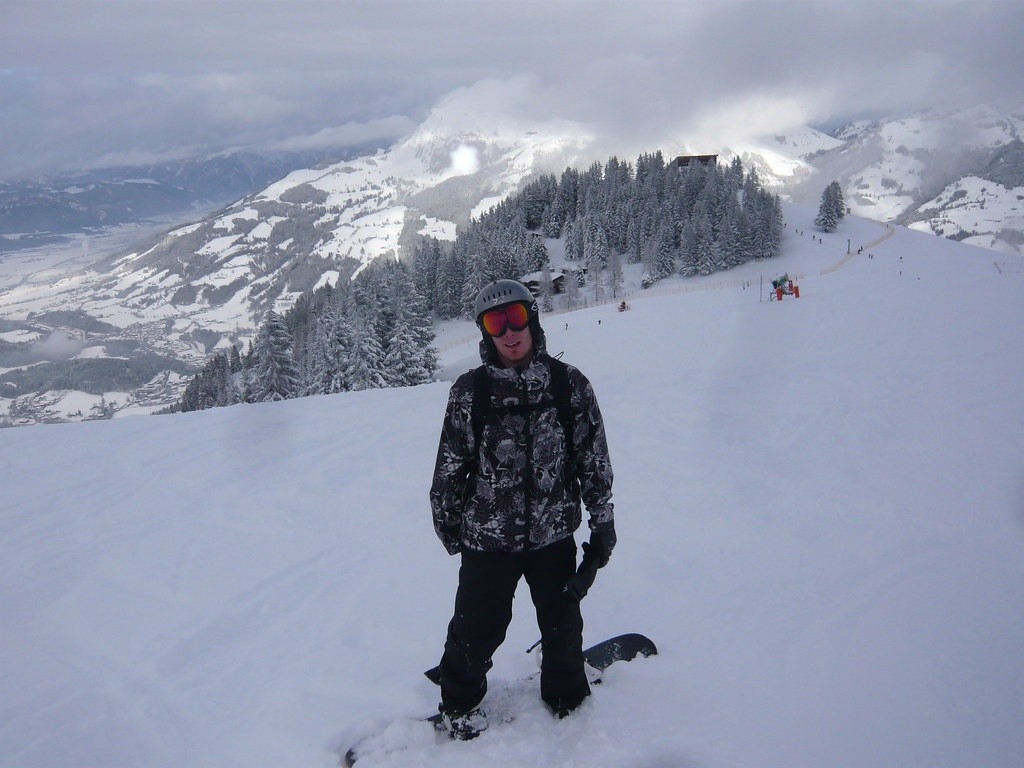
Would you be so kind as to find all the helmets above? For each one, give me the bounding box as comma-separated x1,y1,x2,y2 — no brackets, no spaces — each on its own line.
474,279,539,334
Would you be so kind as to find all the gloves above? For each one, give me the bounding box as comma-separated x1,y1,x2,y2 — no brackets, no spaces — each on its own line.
582,519,617,569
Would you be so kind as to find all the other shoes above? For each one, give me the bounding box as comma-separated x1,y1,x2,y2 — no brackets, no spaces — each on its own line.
543,695,590,720
439,702,489,740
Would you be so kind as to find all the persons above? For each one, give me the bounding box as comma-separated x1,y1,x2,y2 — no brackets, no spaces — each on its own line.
429,280,616,739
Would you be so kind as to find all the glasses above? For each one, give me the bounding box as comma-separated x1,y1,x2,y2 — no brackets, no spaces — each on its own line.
475,299,539,337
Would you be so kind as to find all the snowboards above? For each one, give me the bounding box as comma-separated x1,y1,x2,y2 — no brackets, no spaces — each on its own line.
344,629,661,768
